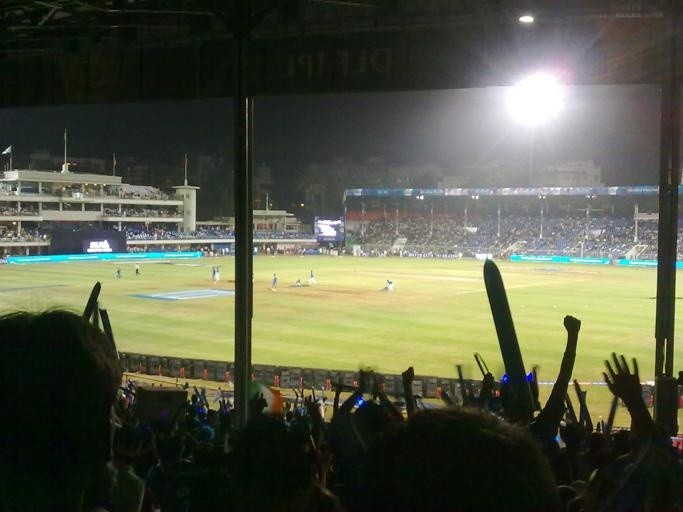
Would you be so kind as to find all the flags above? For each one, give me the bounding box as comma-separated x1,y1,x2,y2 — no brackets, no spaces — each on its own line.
2,145,11,155
